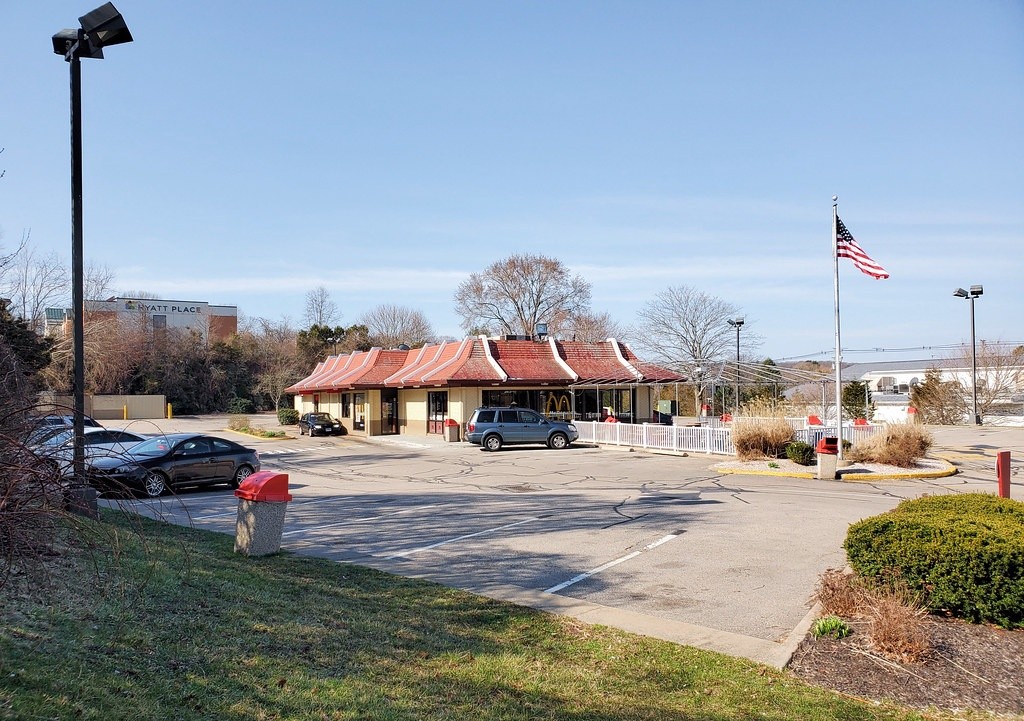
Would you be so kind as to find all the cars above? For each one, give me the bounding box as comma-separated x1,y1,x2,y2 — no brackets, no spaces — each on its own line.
25,411,100,447
22,426,165,483
84,433,263,499
297,411,343,437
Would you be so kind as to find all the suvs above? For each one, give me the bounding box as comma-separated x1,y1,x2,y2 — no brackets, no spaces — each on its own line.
462,402,580,452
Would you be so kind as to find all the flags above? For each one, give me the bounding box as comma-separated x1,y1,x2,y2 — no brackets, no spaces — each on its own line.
836,215,889,280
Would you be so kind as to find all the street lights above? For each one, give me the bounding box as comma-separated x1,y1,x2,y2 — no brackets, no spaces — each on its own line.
952,284,984,425
725,316,744,418
51,0,135,524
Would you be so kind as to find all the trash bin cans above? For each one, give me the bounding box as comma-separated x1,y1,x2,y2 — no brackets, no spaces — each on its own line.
445,419,460,443
907,407,919,426
808,416,823,437
606,417,620,440
702,405,712,417
816,437,838,481
720,414,733,428
234,472,292,556
854,419,869,441
